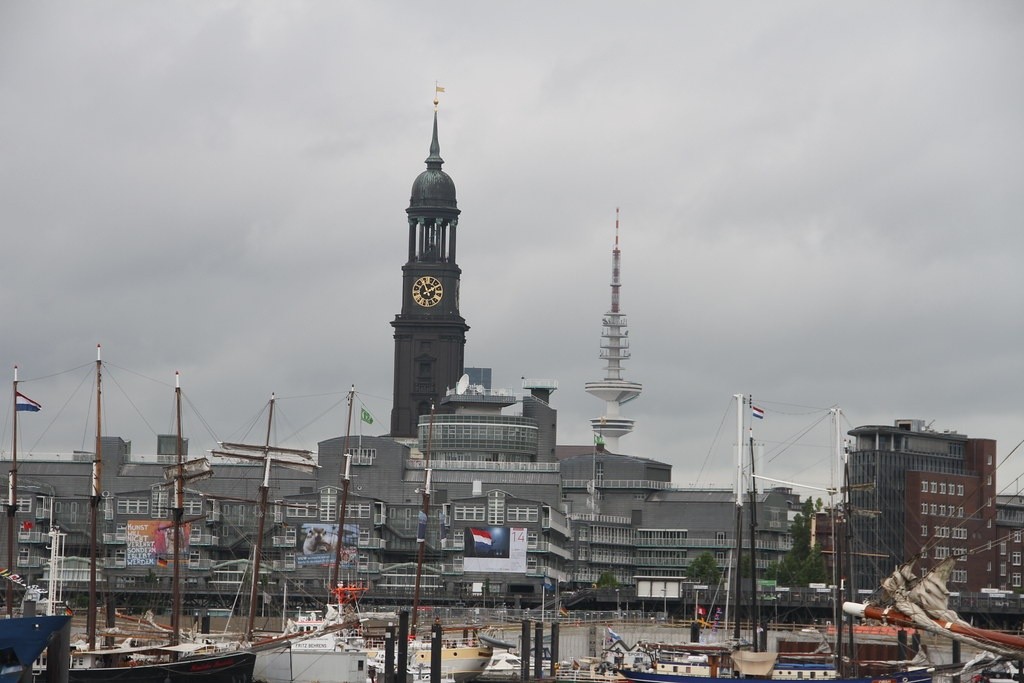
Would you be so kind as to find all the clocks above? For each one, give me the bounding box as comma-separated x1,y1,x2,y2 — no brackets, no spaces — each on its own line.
412,277,443,307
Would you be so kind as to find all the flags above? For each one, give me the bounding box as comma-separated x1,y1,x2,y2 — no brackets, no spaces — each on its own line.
472,529,493,549
752,407,764,419
16,392,41,412
560,606,568,616
361,408,373,424
697,606,706,615
0,567,48,594
608,626,622,641
158,557,168,567
65,605,74,615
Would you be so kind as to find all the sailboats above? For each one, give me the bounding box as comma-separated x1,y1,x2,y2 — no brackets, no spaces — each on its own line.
580,390,1024,683
0,343,574,683
